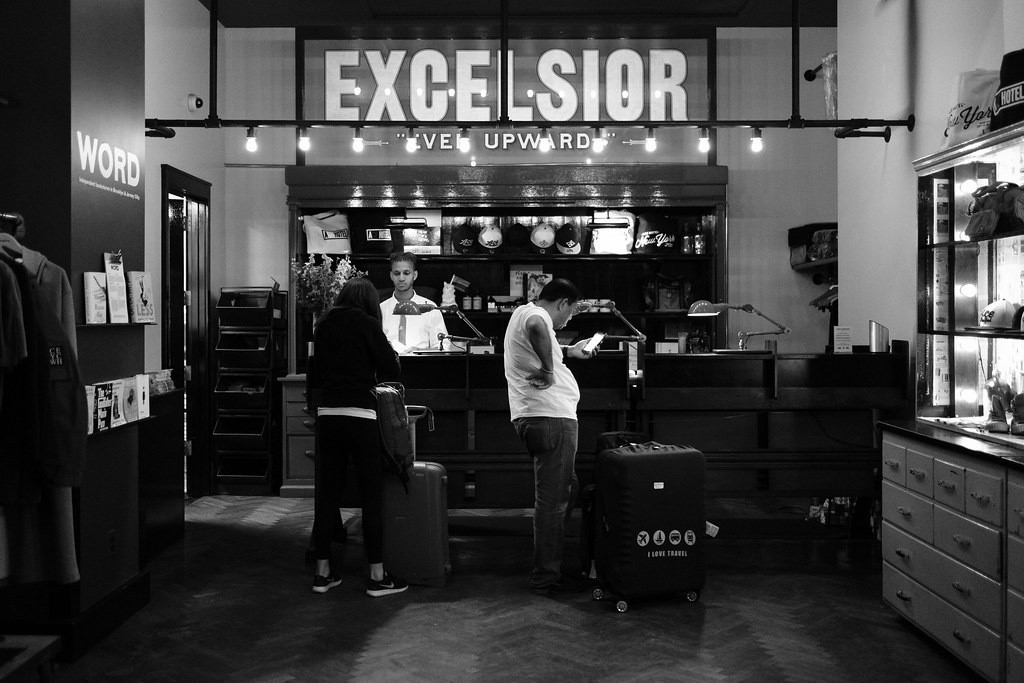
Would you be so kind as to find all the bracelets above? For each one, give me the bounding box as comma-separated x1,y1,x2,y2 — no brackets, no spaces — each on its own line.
540,366,554,374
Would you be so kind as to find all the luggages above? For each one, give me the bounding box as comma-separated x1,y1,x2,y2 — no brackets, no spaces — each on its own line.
593,442,710,612
380,403,452,586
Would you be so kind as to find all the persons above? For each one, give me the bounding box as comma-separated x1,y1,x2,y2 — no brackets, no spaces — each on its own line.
378,252,451,353
505,279,603,593
304,274,408,597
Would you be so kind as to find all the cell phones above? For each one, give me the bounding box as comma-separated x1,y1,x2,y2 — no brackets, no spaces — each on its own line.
582,332,608,355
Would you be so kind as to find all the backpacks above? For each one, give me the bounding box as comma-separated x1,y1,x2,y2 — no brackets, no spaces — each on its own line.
369,379,414,494
1,248,89,476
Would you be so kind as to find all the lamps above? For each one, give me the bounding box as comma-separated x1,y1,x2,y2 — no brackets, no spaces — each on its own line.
573,301,647,354
392,301,493,354
686,301,789,349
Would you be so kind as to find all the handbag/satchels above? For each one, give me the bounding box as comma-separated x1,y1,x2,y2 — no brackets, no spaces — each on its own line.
965,181,1024,237
989,48,1024,130
945,67,1000,147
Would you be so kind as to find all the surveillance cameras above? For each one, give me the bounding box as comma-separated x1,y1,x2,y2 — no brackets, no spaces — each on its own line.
188,94,203,112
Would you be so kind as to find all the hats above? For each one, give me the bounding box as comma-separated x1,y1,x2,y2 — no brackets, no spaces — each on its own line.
963,299,1021,331
995,306,1024,334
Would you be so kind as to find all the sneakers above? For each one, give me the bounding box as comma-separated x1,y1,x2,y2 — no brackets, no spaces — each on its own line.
367,573,409,598
311,570,341,592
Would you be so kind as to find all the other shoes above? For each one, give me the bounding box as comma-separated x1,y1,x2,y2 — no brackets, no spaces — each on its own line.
531,572,592,597
983,395,1009,433
1010,393,1024,435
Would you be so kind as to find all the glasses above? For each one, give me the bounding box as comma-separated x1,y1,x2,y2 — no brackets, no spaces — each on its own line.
566,301,579,316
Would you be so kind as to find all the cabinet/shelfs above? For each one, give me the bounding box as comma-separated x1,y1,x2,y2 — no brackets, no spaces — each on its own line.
210,285,277,494
881,433,1024,683
284,165,728,355
908,125,1024,450
280,383,316,498
